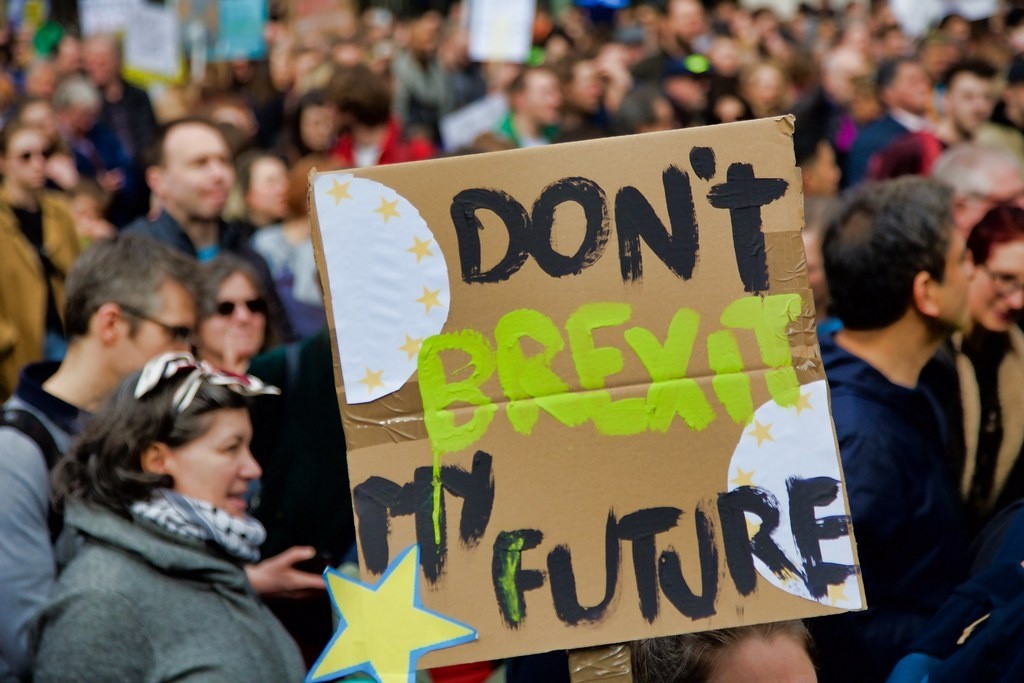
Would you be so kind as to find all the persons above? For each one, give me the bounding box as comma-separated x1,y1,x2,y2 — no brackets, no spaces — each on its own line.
31,348,309,683
0,235,324,683
0,0,1024,683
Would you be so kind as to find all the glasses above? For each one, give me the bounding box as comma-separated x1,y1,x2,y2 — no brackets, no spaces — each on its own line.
217,297,268,315
5,146,54,164
976,264,1024,295
119,304,199,356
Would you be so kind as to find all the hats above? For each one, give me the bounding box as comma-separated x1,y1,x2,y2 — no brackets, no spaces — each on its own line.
659,56,714,75
